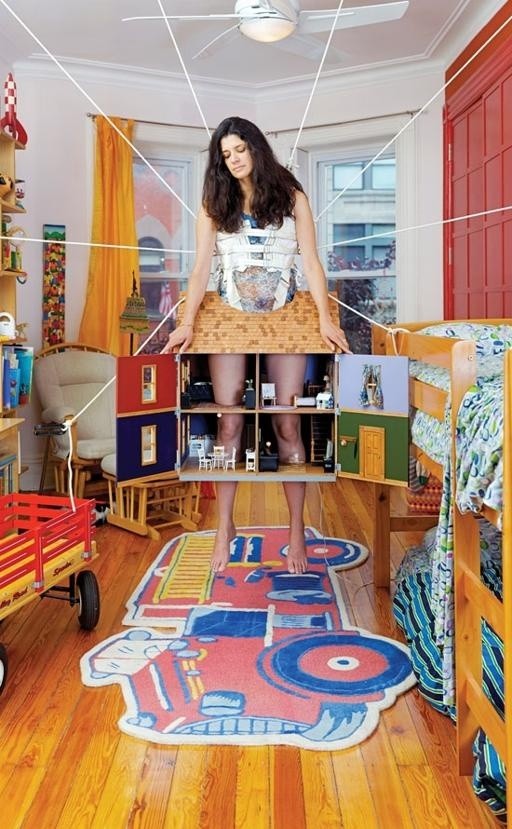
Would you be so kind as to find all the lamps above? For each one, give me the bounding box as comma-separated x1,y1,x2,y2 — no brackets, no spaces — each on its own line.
120,267,150,357
239,16,296,44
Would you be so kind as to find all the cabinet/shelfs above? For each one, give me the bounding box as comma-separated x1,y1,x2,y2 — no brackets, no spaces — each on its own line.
0,128,27,498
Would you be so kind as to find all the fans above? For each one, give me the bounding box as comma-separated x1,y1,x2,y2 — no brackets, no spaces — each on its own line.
122,0,409,62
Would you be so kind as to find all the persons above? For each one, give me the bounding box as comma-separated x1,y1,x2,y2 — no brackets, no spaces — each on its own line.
160,113,355,573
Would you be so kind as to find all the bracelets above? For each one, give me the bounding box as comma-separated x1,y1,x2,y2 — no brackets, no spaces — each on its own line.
178,323,194,327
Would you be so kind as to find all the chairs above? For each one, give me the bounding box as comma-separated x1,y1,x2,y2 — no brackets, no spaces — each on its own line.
30,340,117,517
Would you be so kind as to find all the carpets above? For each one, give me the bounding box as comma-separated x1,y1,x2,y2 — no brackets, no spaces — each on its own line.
79,524,419,753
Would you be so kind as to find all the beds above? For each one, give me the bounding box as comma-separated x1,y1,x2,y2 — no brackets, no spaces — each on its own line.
363,315,512,829
